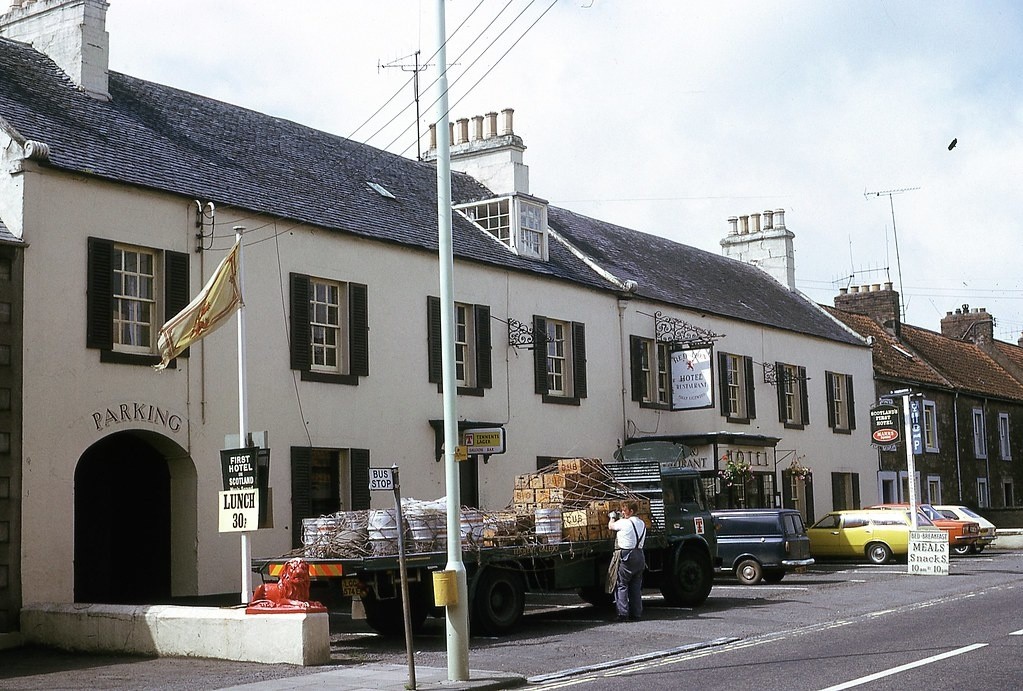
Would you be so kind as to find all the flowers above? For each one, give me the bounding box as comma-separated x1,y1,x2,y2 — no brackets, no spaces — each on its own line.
717,456,755,487
786,454,813,481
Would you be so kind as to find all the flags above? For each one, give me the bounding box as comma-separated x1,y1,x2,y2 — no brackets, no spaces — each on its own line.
153,235,246,373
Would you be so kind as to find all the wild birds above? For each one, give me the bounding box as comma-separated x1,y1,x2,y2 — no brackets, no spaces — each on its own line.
948,138,957,150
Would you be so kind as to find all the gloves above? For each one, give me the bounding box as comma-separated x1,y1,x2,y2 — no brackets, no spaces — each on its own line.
608,511,616,518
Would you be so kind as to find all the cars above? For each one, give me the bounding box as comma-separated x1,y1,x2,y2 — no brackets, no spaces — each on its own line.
862,502,982,549
922,505,998,556
805,509,939,565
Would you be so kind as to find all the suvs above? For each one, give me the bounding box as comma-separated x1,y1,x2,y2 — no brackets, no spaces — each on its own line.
709,508,815,586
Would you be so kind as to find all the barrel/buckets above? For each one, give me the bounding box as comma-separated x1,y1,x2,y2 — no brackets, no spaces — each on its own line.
296,504,483,556
535,507,562,545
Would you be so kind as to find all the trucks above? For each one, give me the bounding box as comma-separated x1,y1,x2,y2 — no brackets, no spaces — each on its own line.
250,459,724,642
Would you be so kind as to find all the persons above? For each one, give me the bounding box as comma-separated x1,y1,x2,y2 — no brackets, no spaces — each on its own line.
608,501,646,623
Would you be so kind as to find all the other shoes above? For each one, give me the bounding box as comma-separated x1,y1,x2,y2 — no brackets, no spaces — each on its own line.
631,616,642,621
609,616,631,622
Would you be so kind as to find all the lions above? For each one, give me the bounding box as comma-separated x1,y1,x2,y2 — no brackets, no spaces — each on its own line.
249,557,322,608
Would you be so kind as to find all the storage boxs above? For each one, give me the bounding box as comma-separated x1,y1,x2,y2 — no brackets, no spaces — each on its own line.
482,458,650,547
